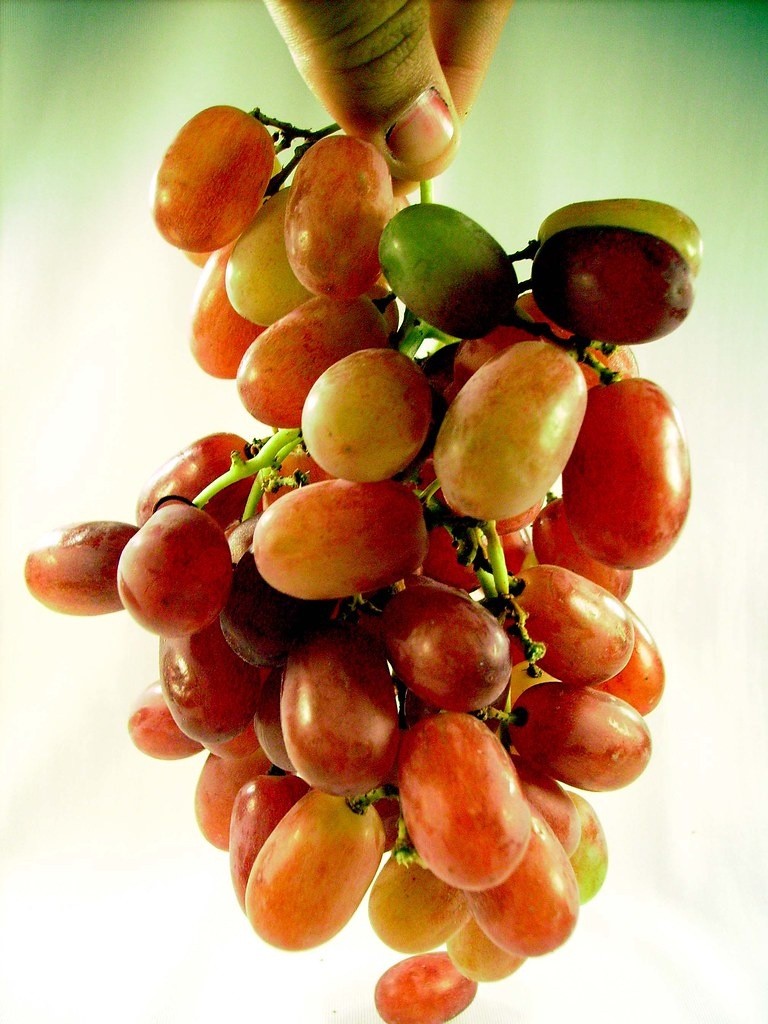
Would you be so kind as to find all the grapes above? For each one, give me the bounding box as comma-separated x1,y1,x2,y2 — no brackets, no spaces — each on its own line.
19,107,702,1024
